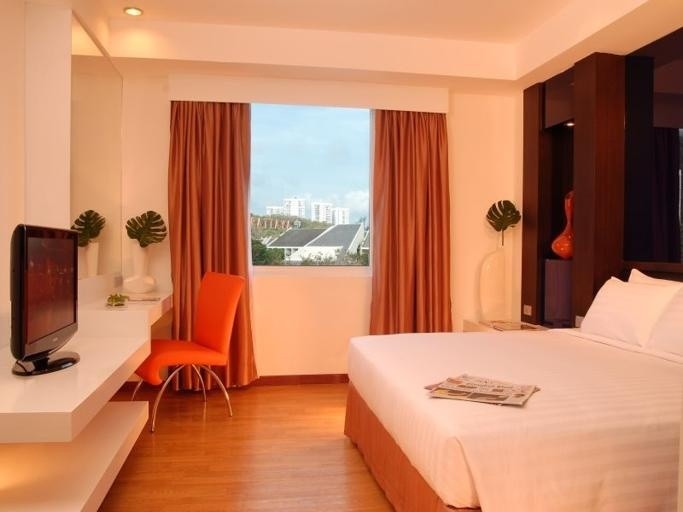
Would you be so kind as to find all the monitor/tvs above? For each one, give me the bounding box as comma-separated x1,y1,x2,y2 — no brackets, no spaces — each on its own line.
9,223,81,376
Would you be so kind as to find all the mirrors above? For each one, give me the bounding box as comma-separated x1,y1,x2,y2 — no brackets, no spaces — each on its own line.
70,10,123,305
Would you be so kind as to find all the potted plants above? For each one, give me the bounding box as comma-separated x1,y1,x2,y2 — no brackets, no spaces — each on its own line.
123,211,168,294
479,200,521,320
71,210,106,290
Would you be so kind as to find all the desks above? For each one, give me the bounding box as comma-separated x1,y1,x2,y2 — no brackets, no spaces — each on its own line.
0,336,151,444
78,295,173,326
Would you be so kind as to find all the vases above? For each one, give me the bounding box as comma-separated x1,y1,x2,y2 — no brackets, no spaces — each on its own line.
551,192,573,257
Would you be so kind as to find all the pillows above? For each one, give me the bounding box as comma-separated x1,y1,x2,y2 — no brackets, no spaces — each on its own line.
580,277,683,347
628,269,683,358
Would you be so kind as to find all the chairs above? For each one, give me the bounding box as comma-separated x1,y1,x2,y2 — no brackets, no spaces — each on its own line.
131,272,246,433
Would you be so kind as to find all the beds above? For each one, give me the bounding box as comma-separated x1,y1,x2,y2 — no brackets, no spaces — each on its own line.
344,262,683,512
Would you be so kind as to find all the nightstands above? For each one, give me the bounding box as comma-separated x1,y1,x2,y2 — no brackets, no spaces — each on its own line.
463,320,549,332
